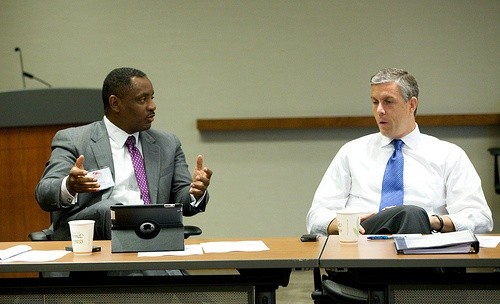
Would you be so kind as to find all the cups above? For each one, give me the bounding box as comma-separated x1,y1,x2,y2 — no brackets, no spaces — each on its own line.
68,220,95,255
336,210,360,244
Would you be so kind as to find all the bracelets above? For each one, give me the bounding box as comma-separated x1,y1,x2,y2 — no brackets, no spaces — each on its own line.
432,215,444,233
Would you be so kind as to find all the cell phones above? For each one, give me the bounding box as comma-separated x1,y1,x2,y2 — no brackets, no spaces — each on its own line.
65,245,101,253
300,235,317,242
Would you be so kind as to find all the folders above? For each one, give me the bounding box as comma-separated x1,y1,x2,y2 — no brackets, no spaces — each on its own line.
394,230,479,255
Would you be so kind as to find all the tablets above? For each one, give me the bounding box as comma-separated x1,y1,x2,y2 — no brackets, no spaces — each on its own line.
110,203,184,252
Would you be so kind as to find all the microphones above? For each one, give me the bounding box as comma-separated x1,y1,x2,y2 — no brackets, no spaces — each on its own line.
14,47,51,89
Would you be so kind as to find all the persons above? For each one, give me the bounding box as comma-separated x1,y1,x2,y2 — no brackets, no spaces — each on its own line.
306,69,494,278
35,69,213,278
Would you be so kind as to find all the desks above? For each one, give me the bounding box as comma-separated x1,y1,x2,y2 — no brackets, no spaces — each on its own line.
0,234,328,304
319,233,500,304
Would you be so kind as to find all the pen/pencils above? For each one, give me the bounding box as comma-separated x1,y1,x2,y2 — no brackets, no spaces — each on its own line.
365,236,408,239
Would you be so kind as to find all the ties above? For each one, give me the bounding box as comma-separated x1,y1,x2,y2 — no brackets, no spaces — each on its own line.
379,139,405,213
124,135,151,205
